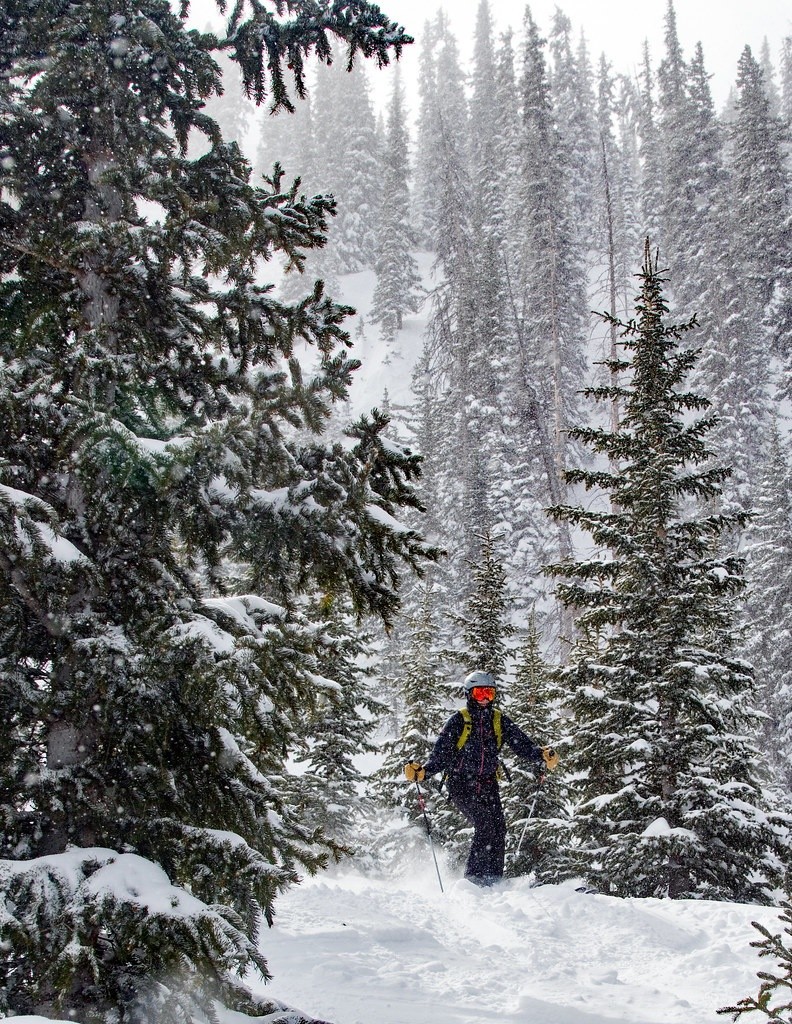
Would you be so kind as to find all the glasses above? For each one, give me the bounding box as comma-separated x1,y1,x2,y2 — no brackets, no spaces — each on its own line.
471,688,496,701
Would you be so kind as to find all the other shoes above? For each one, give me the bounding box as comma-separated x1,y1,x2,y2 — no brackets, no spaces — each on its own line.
463,872,491,888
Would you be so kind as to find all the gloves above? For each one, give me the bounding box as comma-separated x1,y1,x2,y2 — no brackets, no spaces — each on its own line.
405,762,425,782
544,748,559,769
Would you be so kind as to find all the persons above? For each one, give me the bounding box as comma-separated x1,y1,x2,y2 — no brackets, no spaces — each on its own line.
405,671,559,887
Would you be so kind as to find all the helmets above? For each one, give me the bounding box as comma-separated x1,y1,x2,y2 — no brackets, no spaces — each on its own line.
463,671,497,695
